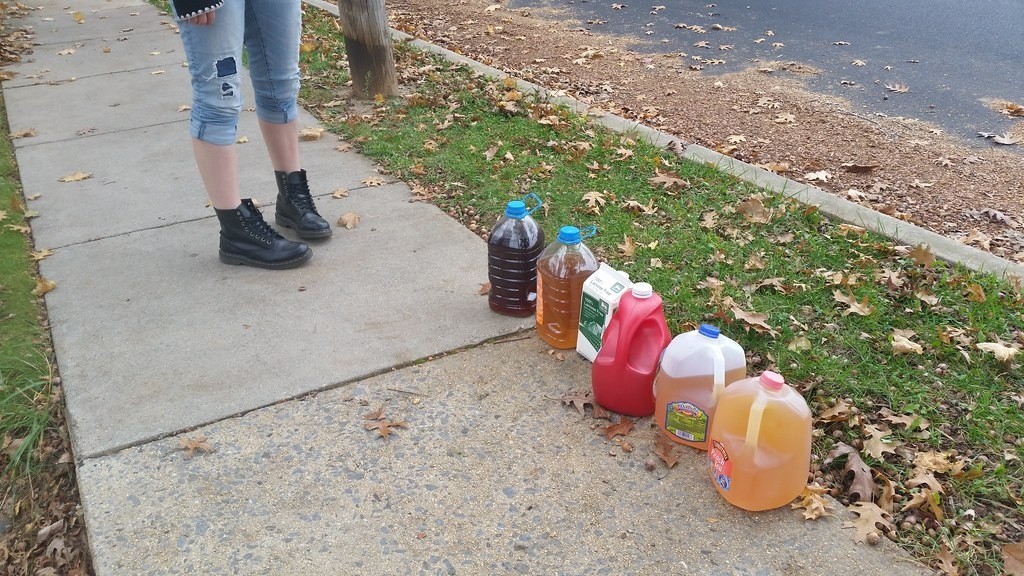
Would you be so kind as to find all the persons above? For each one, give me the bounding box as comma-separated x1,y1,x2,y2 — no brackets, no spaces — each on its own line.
169,0,332,270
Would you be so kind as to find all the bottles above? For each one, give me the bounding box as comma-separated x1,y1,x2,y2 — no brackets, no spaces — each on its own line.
591,281,672,417
707,370,814,512
535,226,598,349
653,324,747,450
486,194,544,318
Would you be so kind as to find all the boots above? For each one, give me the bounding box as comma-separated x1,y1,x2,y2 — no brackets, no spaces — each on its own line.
274,169,332,239
214,198,314,269
